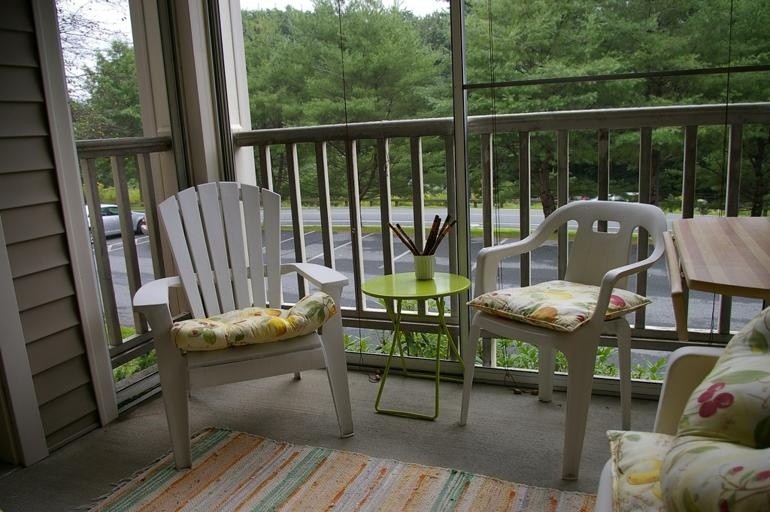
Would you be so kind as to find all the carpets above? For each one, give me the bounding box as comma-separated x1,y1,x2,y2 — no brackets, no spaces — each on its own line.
71,425,594,512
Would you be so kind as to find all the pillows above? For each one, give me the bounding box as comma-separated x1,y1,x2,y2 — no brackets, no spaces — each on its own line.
660,303,770,509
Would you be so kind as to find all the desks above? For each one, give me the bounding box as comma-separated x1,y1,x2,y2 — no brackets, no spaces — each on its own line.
360,272,473,422
667,216,770,301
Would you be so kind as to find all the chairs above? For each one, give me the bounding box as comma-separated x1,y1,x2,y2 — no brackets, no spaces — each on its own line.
457,199,670,482
128,182,357,471
594,346,770,511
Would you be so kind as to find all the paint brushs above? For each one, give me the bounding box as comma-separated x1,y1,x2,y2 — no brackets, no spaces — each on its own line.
386,214,457,256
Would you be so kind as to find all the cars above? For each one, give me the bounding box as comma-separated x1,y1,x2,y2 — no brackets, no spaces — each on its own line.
85,203,146,243
